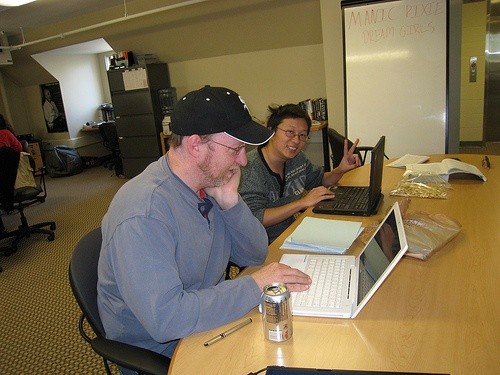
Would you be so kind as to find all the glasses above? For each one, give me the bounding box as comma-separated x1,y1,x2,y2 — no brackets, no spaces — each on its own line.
208,139,245,155
480,156,490,168
277,127,309,142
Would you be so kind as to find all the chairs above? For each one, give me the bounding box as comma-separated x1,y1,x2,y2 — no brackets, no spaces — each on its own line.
99,122,123,176
0,140,55,271
328,127,375,168
69,227,172,375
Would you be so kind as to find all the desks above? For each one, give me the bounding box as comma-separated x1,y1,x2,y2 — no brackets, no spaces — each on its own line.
81,128,98,131
160,118,331,173
169,153,500,375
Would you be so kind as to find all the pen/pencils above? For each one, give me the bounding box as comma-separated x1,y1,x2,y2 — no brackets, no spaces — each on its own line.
204,318,252,346
328,185,332,189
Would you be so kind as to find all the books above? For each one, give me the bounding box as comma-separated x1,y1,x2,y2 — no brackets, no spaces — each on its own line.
406,158,487,184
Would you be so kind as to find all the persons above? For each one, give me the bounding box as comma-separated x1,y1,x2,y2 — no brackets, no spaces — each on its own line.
96,83,312,375
43,90,59,130
238,103,361,245
0,113,23,216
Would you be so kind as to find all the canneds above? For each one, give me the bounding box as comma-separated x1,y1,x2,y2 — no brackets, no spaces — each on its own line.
261,283,293,343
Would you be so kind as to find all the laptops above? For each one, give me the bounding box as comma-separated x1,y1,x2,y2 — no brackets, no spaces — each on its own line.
258,201,408,318
312,136,385,215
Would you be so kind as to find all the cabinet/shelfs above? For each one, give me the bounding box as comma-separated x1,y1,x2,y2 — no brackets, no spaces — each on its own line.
105,62,169,179
27,143,44,176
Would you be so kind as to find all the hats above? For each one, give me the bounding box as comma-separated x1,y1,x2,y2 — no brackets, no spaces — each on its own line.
168,85,275,145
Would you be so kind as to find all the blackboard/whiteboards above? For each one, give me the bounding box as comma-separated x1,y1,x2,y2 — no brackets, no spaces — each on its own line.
344,0,446,163
